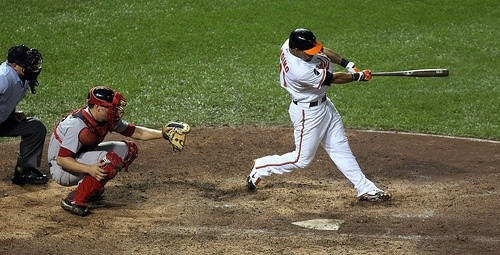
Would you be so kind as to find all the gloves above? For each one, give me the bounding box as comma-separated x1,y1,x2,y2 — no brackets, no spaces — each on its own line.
340,58,361,74
351,69,373,83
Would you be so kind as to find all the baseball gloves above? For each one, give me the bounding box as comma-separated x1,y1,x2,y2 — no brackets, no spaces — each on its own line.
162,122,191,154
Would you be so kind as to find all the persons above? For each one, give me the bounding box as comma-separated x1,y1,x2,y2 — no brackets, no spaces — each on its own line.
47,85,192,216
245,27,395,202
0,45,50,186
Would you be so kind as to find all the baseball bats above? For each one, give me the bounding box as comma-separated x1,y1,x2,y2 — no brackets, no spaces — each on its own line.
371,68,450,77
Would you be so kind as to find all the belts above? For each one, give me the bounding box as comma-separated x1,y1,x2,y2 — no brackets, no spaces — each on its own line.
292,92,327,107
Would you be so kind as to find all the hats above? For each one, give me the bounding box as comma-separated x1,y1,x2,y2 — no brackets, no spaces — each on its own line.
288,28,323,55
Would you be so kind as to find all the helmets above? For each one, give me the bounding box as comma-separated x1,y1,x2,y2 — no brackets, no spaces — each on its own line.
7,44,43,94
86,85,127,134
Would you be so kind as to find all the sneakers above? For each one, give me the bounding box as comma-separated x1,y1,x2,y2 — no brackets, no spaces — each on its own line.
358,188,393,203
11,165,50,186
88,193,105,202
244,158,263,195
61,195,88,216
